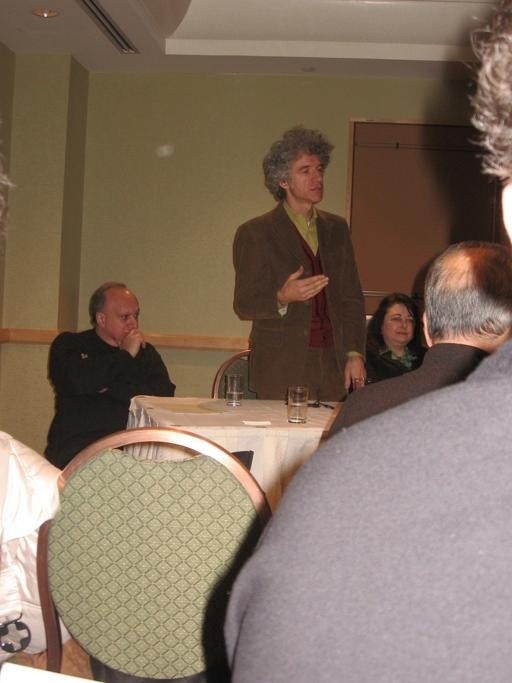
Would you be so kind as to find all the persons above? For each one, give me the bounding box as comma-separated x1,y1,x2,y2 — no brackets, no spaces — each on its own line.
214,1,511,683
363,291,428,382
231,124,368,404
328,237,511,449
45,280,177,467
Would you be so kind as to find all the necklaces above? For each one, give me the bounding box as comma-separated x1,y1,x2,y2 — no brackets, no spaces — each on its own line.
302,206,313,234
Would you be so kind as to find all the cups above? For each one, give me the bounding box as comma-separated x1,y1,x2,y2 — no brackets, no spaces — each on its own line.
289,386,308,424
225,373,244,405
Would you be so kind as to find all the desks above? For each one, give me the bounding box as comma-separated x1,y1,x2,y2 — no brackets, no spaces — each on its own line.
122,394,346,515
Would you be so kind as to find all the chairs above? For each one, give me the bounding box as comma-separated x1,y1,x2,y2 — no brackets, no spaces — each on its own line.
212,350,250,399
37,426,272,683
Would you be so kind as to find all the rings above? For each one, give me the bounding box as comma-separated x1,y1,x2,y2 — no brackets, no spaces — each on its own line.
353,377,361,383
